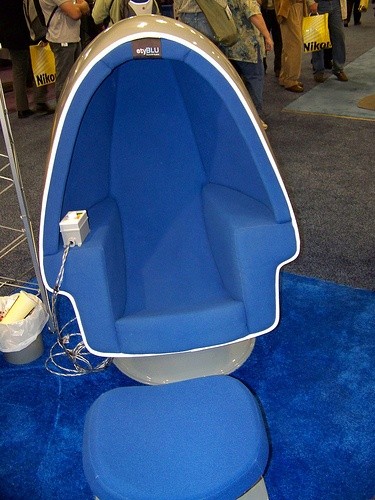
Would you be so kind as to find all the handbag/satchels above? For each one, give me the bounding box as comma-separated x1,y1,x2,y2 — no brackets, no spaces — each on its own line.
29,40,56,88
302,12,332,53
358,0,369,12
196,0,240,47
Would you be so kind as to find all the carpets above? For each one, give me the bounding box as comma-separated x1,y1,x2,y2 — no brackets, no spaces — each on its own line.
0,262,374,500
280,45,375,121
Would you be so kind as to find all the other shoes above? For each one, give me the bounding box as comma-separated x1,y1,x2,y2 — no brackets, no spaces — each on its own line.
275,71,280,76
332,70,349,80
344,20,348,27
18,109,33,118
280,81,304,87
313,71,325,82
285,84,304,93
264,68,267,74
37,103,49,112
311,58,333,69
354,21,361,25
260,119,268,130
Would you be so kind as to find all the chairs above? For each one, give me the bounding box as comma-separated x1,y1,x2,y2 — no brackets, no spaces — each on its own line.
36,18,301,386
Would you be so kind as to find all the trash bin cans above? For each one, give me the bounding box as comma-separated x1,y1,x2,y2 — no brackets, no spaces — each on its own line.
1,291,45,366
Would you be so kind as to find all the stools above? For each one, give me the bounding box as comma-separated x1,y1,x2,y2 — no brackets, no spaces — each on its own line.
83,376,270,500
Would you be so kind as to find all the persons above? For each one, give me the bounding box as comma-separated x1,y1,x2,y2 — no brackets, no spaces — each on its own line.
0,0,375,130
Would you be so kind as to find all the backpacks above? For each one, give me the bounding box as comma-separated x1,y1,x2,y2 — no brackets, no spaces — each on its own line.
23,0,59,44
120,0,156,19
